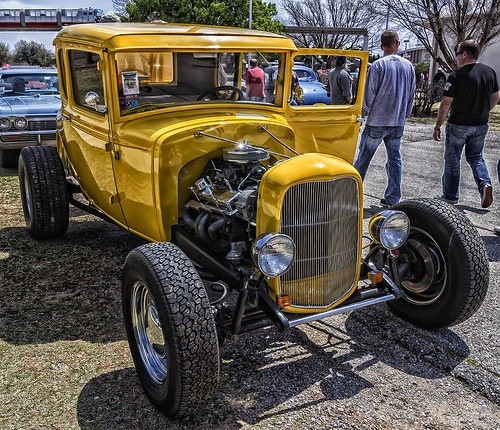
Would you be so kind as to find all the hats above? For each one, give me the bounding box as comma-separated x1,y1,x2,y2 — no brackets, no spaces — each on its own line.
12,78,28,85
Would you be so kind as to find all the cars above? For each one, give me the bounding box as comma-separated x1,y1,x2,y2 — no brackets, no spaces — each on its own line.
226,75,246,91
18,22,490,419
264,65,330,105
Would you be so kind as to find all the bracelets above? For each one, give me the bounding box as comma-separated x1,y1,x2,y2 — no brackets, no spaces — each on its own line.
434,127,440,131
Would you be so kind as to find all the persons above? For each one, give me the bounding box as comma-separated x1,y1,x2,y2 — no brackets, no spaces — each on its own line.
245,59,266,102
354,29,416,205
273,70,299,105
433,41,500,208
320,57,353,105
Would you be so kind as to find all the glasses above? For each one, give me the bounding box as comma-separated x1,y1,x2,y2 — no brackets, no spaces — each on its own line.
456,51,467,58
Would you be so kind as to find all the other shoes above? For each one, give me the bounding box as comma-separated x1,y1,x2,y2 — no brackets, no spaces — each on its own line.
434,194,458,204
494,227,500,234
380,199,392,209
480,185,495,208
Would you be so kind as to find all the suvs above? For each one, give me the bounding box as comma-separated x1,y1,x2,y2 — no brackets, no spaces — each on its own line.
0,65,62,168
